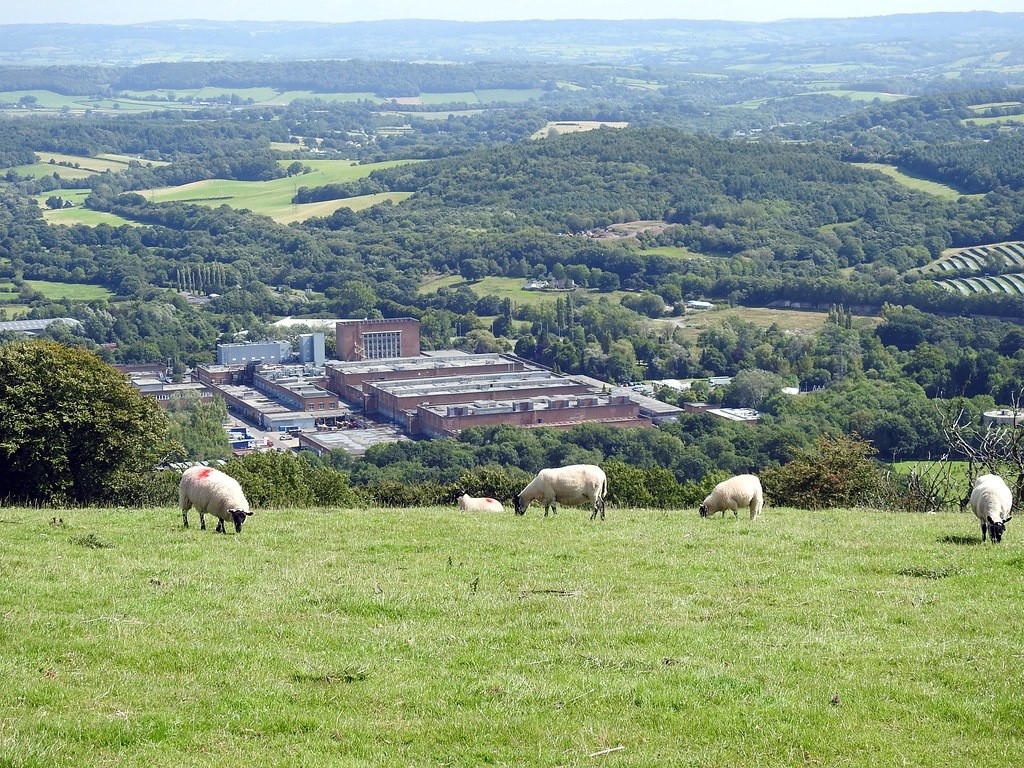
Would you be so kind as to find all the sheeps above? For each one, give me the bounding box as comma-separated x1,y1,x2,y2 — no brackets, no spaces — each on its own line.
178,465,254,534
699,474,764,522
971,474,1013,544
512,463,610,521
457,493,505,513
